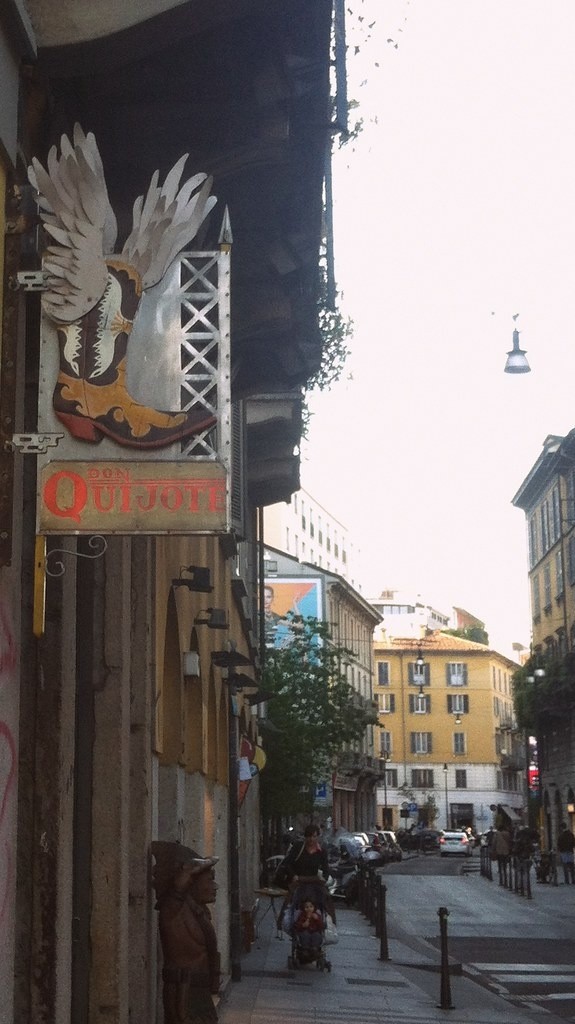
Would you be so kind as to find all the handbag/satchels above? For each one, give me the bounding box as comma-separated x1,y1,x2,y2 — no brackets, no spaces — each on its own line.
275,841,305,880
491,849,497,861
281,905,302,938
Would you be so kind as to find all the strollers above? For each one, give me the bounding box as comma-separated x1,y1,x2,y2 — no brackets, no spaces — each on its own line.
286,879,332,971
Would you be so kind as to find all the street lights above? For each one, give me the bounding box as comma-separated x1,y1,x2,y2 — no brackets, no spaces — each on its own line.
379,750,391,830
443,763,449,829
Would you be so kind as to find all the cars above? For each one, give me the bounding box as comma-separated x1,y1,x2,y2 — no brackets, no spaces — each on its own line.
395,828,444,850
325,828,401,876
439,833,473,857
467,833,475,848
480,830,496,847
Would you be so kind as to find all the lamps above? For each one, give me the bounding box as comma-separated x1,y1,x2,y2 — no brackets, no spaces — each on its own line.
417,650,424,665
418,685,425,699
211,650,254,667
193,608,230,630
243,690,277,706
173,565,214,593
504,328,531,373
221,673,259,692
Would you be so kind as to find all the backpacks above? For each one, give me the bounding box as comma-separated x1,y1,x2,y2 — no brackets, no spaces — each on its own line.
323,913,339,945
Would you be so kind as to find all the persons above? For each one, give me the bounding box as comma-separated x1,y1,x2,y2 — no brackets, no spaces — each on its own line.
535,854,551,883
151,841,221,1024
295,900,324,952
485,824,540,874
558,823,575,884
258,586,304,648
287,824,330,883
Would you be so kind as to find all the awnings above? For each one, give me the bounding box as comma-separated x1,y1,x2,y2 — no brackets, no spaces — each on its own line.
502,806,521,822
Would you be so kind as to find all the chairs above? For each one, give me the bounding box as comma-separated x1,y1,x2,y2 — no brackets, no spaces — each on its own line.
254,886,289,941
